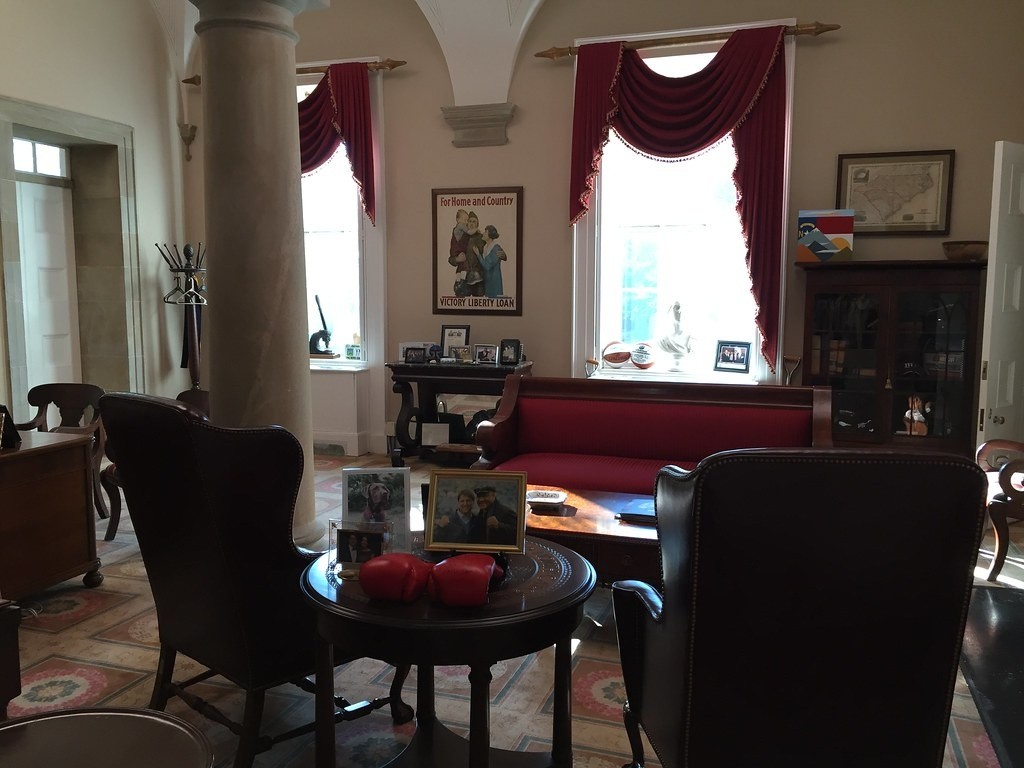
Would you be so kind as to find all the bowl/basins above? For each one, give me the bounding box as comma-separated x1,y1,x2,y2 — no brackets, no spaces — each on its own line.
526,489,568,509
942,240,989,262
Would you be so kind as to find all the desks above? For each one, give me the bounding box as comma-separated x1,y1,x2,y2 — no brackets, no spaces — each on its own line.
525,483,662,593
0,704,214,768
299,534,598,768
384,360,535,467
0,429,105,602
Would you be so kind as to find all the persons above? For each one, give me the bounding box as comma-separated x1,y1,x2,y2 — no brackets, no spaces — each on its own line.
341,534,373,561
503,345,514,359
480,348,495,360
432,485,517,543
453,349,461,359
408,351,422,359
722,350,745,362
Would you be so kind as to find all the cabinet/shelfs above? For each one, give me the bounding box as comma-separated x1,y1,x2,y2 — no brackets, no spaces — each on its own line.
793,259,987,452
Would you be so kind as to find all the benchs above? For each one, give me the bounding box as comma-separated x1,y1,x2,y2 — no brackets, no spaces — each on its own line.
468,372,834,496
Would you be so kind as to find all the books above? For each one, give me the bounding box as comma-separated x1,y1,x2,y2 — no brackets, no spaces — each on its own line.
620,498,655,523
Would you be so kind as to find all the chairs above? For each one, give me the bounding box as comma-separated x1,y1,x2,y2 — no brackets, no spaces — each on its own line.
99,390,210,543
15,382,110,520
611,447,988,768
97,391,415,768
975,438,1024,583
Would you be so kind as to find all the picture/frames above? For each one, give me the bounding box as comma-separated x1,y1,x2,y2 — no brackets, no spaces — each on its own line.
327,519,394,577
341,467,411,554
404,347,427,364
424,469,528,555
499,338,521,365
712,339,753,374
431,186,523,317
835,149,956,237
440,325,470,358
475,345,499,365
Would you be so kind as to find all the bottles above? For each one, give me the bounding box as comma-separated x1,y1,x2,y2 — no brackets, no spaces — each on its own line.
345,344,361,359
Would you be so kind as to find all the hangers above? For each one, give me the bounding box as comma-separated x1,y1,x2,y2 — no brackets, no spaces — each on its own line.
176,277,207,305
188,279,208,303
163,277,193,304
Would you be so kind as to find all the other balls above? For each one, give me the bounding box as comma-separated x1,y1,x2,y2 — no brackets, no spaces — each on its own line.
602,341,630,369
630,343,657,369
